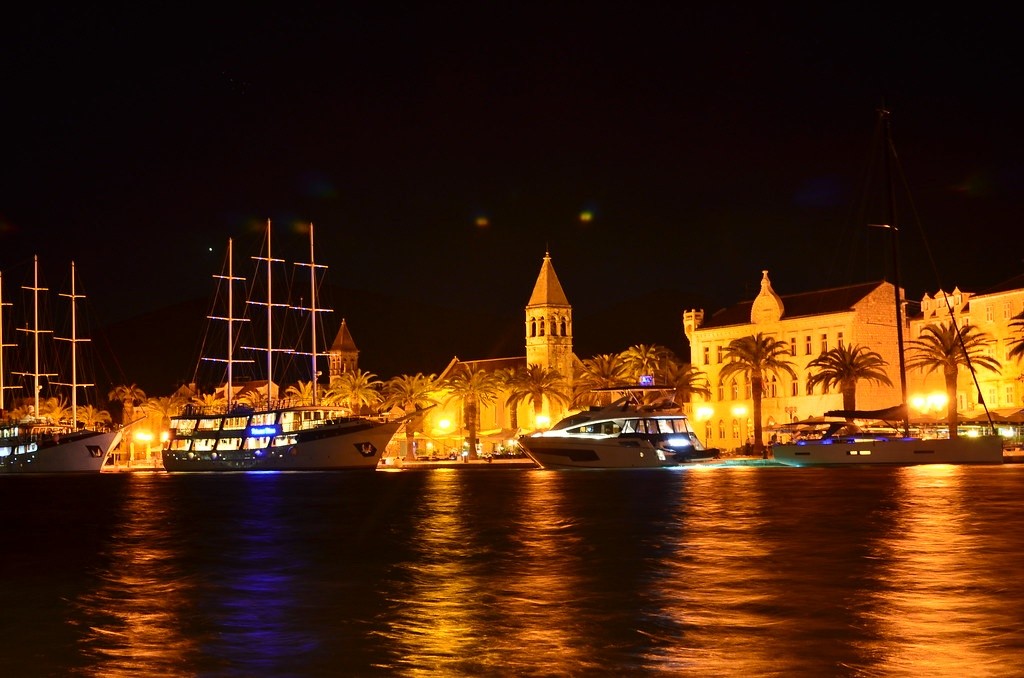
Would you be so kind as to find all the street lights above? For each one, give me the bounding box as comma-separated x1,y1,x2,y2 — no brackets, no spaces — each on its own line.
699,408,712,447
927,394,946,438
734,407,746,448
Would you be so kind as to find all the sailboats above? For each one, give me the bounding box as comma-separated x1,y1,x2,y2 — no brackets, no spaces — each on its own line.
766,223,1008,467
0,253,147,475
151,219,444,469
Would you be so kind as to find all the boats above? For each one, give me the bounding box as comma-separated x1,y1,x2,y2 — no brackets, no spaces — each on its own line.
515,375,725,468
